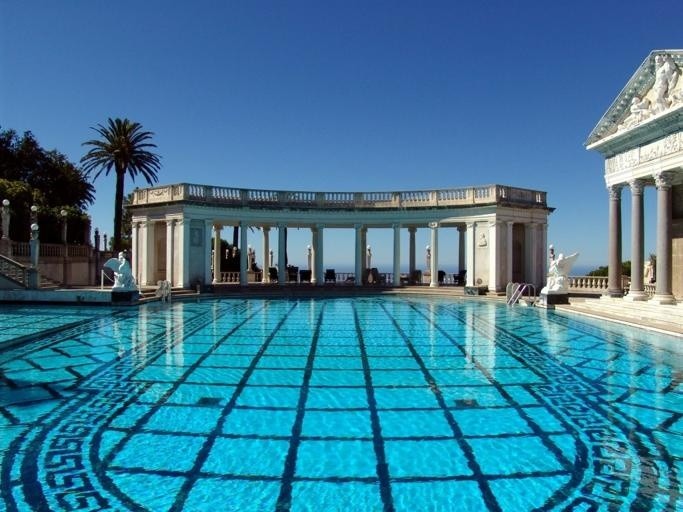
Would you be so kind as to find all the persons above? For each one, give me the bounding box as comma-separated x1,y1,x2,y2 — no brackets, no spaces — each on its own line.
633,55,679,118
545,250,582,290
622,95,649,128
102,250,135,289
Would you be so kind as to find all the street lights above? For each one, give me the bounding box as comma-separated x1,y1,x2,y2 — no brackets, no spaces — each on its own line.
270,249,273,267
247,245,252,270
61,209,67,243
226,247,238,260
549,244,554,263
94,228,109,255
366,244,372,268
425,245,432,272
28,205,40,268
211,249,214,272
2,200,10,239
307,245,312,271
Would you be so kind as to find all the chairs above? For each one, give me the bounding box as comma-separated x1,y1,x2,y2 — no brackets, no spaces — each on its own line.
370,267,386,285
324,269,337,284
268,267,278,282
